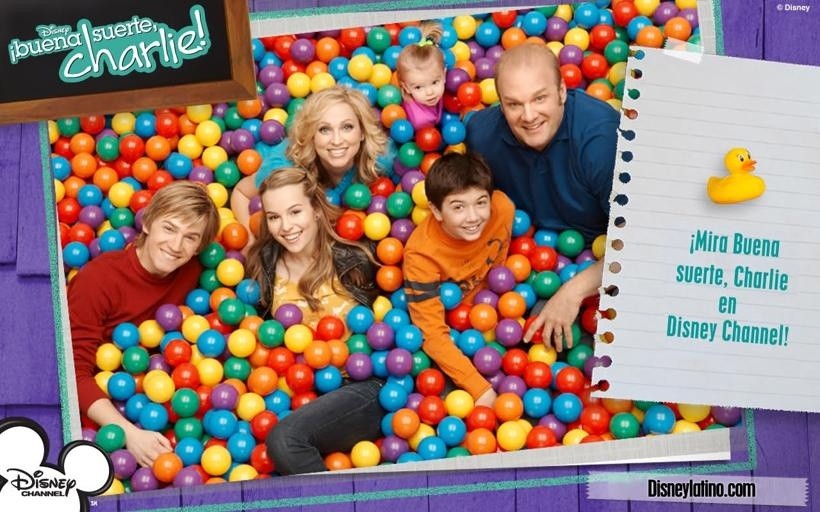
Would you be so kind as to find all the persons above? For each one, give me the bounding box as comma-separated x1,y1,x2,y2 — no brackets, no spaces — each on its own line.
46,2,744,494
